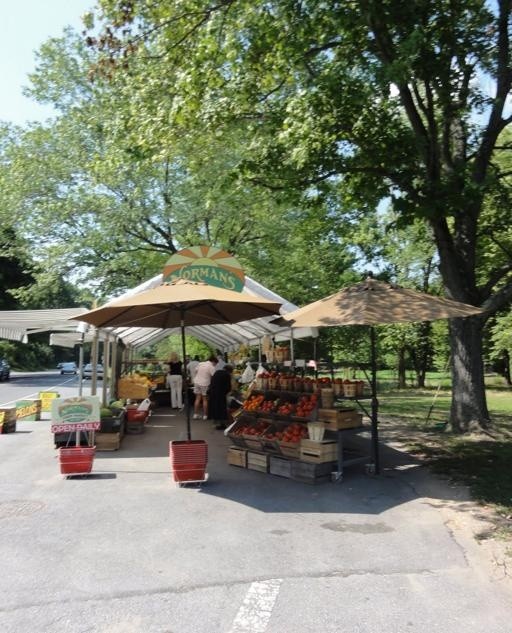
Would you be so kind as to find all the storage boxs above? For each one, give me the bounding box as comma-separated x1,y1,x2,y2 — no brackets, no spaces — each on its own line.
227,393,363,482
90,365,168,450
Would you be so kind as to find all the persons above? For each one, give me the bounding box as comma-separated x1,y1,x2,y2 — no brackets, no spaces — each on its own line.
139,350,270,431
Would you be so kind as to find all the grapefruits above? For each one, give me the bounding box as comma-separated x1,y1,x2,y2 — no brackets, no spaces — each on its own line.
121,374,151,388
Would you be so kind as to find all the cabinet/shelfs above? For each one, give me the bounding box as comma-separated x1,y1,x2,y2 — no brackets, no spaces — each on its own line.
225,376,378,483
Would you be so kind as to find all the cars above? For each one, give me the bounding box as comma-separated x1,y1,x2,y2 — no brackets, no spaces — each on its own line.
82,364,105,379
0,357,10,384
60,362,77,373
56,361,87,368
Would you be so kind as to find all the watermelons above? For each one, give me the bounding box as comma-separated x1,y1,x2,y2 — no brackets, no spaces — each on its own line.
110,401,123,409
101,407,111,417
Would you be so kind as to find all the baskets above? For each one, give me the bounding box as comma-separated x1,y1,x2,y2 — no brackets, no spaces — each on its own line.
58,445,96,473
169,439,208,481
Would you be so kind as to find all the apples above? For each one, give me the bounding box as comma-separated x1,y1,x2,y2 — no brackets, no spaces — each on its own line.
234,371,364,447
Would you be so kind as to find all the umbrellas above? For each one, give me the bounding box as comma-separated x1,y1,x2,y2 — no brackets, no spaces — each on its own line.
269,275,485,475
67,277,284,440
